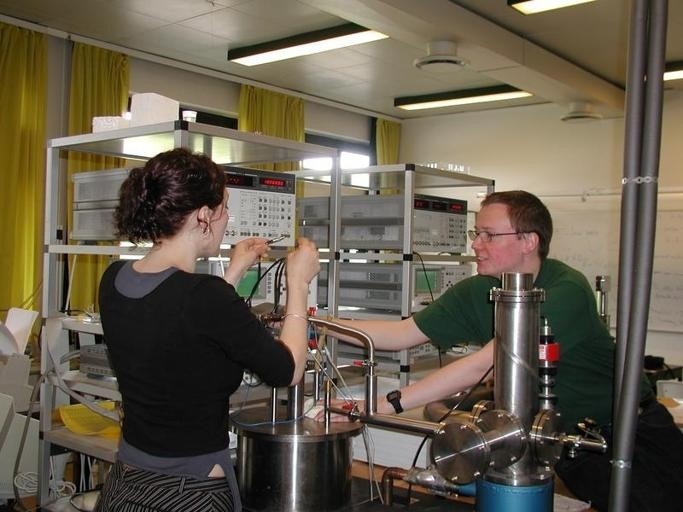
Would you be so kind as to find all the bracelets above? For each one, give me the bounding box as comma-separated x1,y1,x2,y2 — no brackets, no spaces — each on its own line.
279,312,308,323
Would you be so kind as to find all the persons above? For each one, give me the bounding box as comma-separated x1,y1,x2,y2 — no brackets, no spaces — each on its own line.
267,189,682,512
89,145,322,512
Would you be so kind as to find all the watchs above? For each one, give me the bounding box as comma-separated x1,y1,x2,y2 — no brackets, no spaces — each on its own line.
386,390,405,415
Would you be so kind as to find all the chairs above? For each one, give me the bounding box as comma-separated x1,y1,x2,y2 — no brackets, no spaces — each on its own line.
0,305,42,499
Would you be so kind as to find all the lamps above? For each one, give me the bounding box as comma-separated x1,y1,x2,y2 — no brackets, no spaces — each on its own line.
225,23,389,68
660,60,683,82
394,39,535,112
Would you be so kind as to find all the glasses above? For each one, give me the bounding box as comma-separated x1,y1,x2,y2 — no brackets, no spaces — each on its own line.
466,227,536,244
